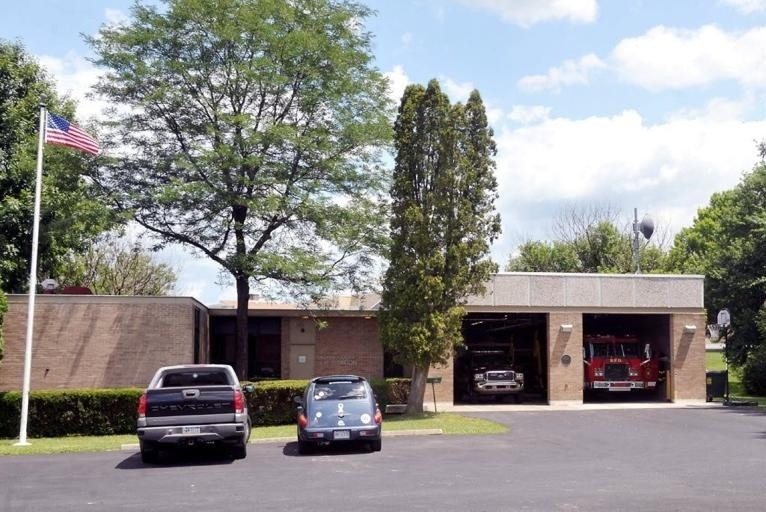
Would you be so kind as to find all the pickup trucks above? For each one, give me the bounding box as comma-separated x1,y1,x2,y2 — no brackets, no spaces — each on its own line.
137,363,252,458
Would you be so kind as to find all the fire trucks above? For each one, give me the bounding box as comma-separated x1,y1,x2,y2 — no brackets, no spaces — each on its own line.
584,335,659,392
454,336,524,402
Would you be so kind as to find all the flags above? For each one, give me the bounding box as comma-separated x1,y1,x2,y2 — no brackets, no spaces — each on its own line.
45,109,99,156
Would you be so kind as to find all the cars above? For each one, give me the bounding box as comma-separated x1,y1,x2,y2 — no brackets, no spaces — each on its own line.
297,374,382,450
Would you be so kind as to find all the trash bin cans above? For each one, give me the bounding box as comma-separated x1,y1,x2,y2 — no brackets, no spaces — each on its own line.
706,370,729,402
